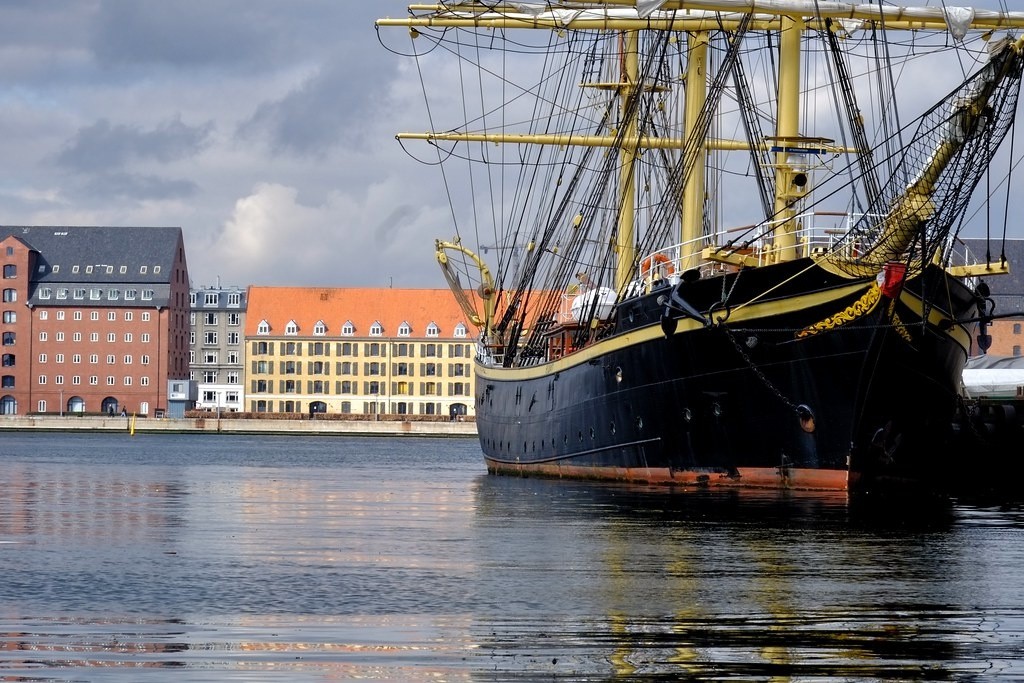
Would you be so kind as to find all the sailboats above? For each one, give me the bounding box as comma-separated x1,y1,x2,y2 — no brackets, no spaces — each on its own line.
374,0,1024,498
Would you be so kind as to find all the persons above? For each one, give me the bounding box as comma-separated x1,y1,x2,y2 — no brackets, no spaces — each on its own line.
108,404,114,417
121,406,127,417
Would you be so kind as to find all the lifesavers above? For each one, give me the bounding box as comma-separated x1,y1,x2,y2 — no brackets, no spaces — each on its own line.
640,254,675,283
